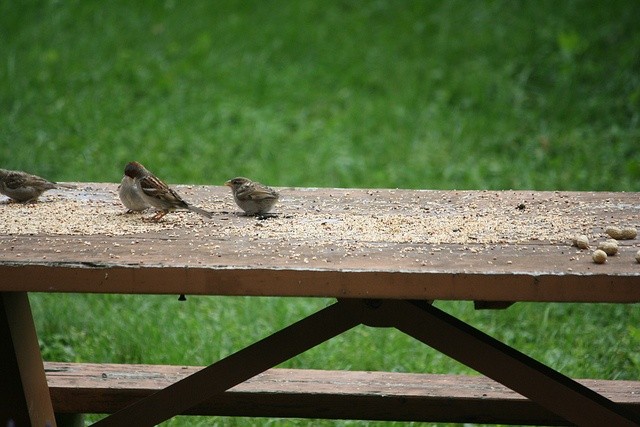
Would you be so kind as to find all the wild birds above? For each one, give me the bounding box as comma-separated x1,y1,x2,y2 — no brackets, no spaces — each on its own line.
224,177,294,217
0,168,77,205
116,172,152,215
125,161,213,223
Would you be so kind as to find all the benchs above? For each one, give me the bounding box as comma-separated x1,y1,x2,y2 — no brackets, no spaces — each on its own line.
40,362,639,426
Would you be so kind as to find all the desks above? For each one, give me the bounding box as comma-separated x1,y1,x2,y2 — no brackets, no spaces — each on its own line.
0,182,639,427
